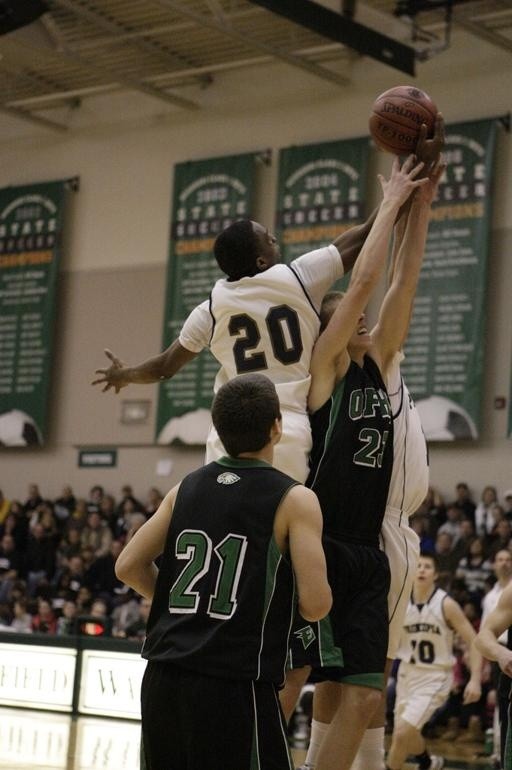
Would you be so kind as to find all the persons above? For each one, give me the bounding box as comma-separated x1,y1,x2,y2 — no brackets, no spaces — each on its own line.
113,371,335,769
299,204,434,770
297,482,512,768
2,482,168,640
275,152,448,770
91,106,448,488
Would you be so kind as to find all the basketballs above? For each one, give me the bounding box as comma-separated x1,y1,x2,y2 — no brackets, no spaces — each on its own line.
369,84,437,156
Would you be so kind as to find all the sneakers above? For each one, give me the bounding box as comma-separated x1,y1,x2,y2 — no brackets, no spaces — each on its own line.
417,755,444,770
